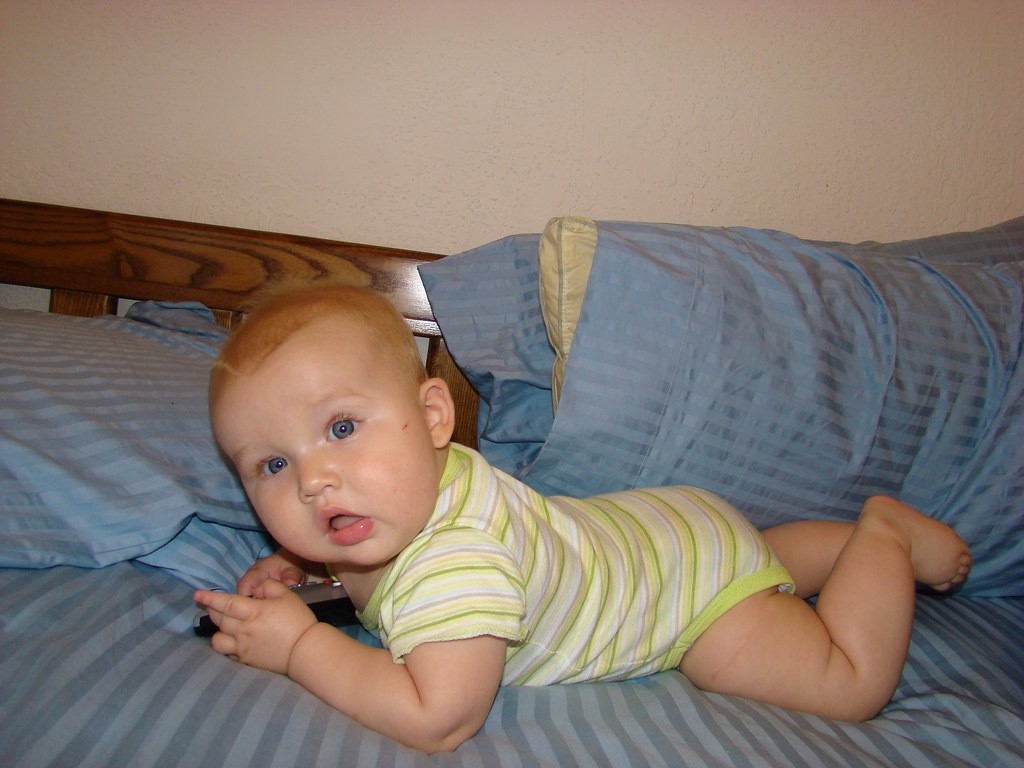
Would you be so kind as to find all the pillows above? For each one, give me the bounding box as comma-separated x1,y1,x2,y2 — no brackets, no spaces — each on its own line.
0,301,283,596
415,217,1024,597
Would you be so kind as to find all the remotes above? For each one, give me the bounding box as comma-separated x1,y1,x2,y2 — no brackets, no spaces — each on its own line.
193,578,359,637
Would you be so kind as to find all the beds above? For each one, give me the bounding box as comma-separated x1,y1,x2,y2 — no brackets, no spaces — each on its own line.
0,196,1024,768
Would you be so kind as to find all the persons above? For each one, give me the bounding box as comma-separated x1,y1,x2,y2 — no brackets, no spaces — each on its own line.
193,288,974,755
